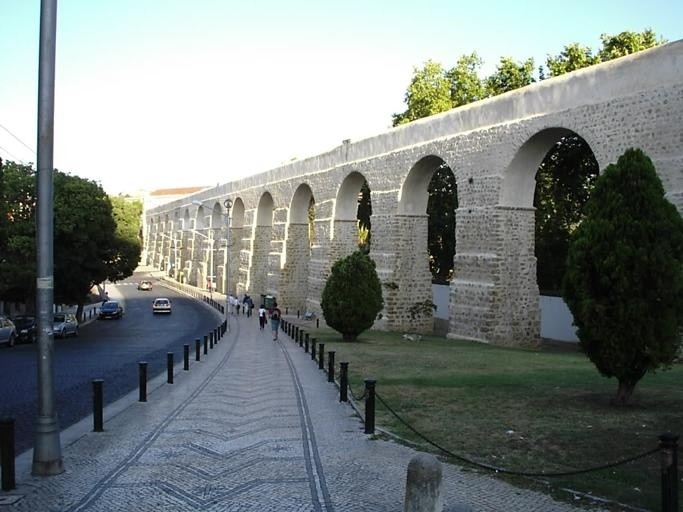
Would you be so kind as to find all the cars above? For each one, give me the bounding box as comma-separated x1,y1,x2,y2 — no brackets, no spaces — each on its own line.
0,313,79,347
169,267,174,277
138,281,152,290
99,301,123,320
151,297,172,315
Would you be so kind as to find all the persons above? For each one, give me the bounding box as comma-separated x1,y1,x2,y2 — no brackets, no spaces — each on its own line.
212,281,216,293
228,293,282,340
208,280,211,292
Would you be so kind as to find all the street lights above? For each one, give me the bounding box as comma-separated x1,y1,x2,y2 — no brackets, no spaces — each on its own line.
191,198,233,316
190,228,215,294
161,233,178,279
